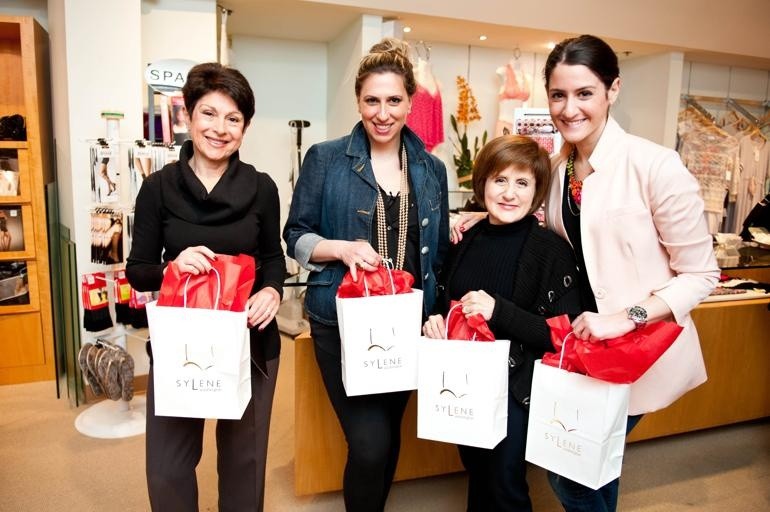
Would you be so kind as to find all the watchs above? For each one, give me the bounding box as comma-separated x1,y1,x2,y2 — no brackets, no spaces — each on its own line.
626,305,651,329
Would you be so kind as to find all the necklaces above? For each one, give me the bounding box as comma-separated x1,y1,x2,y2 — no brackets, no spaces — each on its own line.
564,147,595,217
360,145,411,274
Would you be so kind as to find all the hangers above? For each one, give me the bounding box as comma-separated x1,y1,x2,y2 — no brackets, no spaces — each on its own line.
679,92,770,145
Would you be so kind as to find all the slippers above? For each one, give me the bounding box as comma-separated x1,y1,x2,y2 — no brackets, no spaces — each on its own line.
78,339,135,402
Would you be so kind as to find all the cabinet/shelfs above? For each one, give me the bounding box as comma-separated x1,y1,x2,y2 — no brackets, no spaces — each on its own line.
60,99,168,439
0,17,64,389
293,242,770,498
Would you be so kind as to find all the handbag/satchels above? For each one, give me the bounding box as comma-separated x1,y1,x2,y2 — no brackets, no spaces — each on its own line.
145,254,254,421
524,313,684,490
336,258,511,450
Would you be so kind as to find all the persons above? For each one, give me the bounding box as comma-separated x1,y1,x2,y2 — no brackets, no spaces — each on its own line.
100,158,118,196
136,157,153,181
443,33,726,512
103,220,122,262
0,210,12,251
272,33,452,512
119,60,291,509
489,57,536,140
402,130,590,512
172,106,190,145
